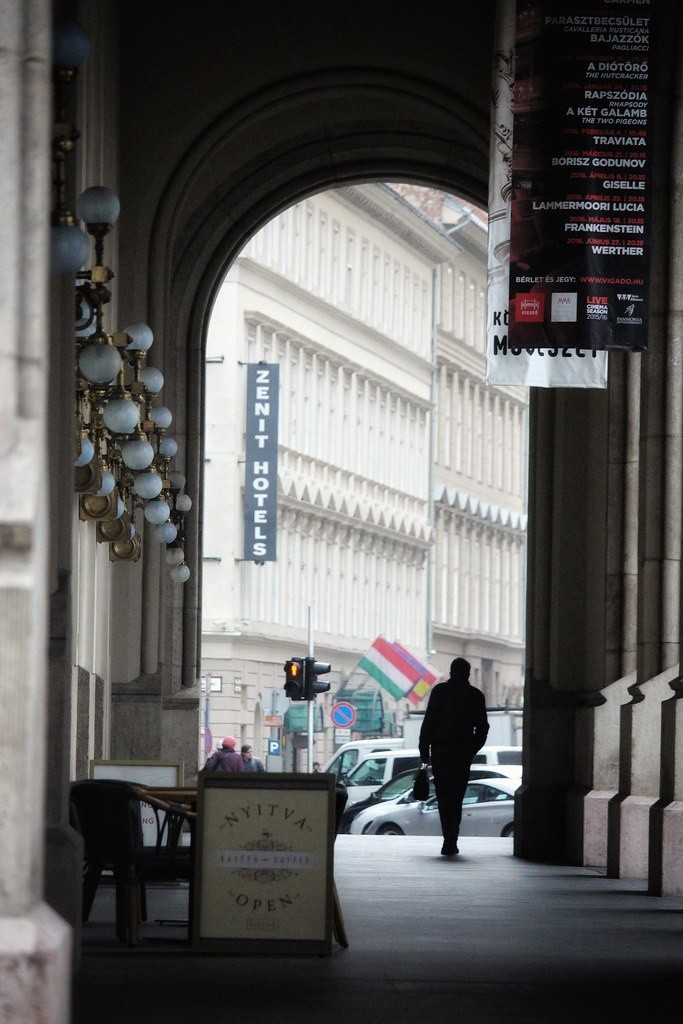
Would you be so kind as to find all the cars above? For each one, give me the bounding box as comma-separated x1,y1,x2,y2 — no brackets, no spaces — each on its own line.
335,768,434,834
361,780,517,838
348,767,522,835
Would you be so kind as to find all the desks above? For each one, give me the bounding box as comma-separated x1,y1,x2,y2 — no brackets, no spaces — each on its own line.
69,776,352,954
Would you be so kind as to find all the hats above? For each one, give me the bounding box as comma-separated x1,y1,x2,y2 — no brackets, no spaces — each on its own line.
221,738,237,749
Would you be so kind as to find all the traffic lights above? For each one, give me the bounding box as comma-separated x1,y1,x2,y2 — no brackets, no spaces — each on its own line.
283,657,305,698
305,657,332,700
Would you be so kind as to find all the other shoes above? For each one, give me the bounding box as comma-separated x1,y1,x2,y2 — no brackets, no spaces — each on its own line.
441,838,462,855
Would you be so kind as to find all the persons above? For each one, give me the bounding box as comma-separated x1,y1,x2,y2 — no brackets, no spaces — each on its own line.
239,744,265,774
419,658,490,857
200,736,244,775
312,762,322,773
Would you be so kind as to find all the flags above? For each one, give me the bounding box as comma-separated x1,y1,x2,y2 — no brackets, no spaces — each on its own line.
392,641,442,706
381,640,425,698
357,636,422,701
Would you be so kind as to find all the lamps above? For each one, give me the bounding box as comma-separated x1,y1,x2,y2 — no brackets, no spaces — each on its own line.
48,121,193,583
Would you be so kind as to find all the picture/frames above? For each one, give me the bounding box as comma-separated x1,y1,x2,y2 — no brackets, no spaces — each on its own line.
89,758,186,850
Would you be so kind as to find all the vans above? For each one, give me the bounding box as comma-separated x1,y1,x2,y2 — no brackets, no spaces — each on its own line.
338,745,522,808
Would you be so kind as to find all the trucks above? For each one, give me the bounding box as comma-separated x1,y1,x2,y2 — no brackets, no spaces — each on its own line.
318,715,512,779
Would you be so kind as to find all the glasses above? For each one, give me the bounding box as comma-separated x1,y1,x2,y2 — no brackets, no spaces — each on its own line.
245,751,253,754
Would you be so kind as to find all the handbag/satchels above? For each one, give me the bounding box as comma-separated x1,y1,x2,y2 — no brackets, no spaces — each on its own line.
412,757,430,801
200,752,232,773
